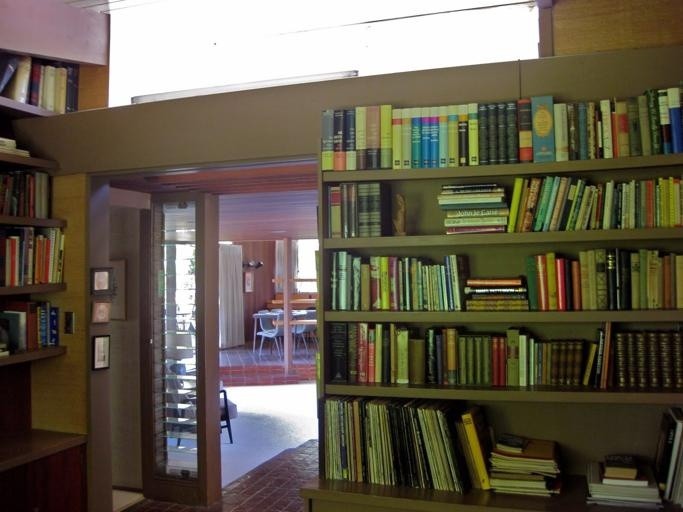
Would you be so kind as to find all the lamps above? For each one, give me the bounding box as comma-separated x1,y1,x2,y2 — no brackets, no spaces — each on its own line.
242,260,264,269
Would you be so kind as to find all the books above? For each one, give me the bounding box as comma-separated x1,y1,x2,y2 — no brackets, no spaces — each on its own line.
1,56,77,359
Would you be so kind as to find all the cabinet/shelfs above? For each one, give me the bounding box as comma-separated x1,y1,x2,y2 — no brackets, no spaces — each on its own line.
0,153,88,512
300,152,683,512
0,0,110,117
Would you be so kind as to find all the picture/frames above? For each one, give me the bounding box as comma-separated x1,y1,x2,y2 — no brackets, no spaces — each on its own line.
92,335,110,370
109,258,128,321
91,299,112,323
243,272,255,293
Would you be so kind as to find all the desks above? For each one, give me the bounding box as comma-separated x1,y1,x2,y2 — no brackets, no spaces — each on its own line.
252,310,317,355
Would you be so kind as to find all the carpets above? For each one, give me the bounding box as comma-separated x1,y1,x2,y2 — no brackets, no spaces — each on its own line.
112,490,145,512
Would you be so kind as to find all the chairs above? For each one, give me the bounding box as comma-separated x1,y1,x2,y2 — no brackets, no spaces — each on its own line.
257,313,293,361
165,362,238,447
271,309,283,312
258,310,283,352
292,311,319,360
